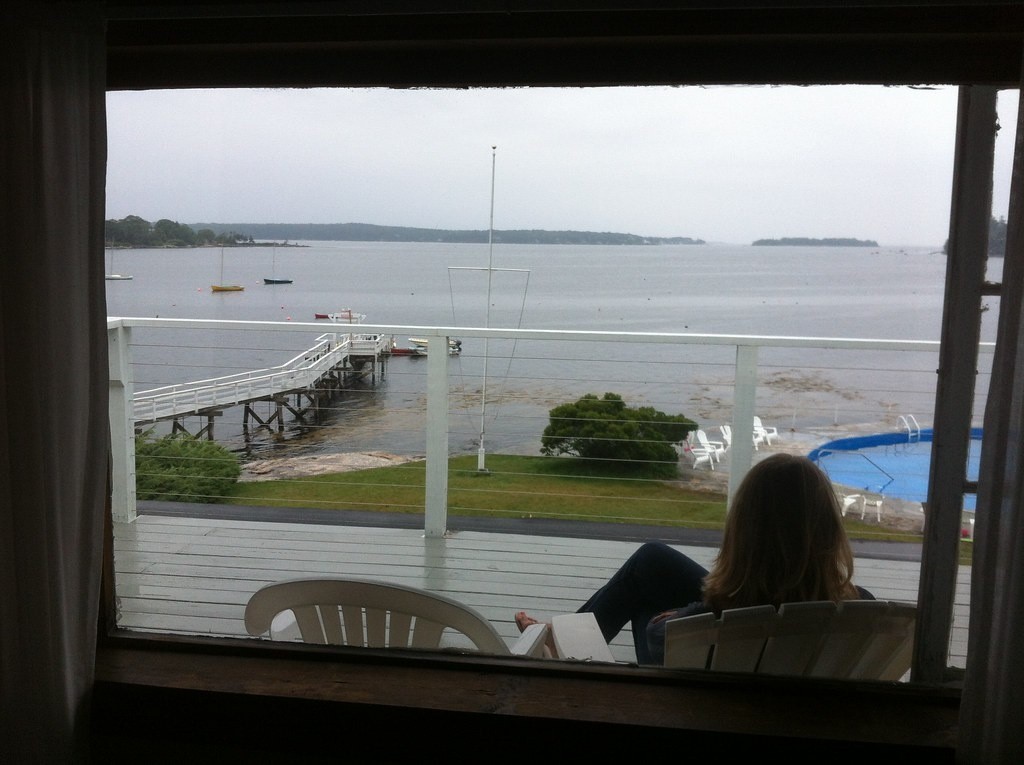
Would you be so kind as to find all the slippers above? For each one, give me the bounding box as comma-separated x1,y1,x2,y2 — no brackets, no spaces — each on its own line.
514,611,556,660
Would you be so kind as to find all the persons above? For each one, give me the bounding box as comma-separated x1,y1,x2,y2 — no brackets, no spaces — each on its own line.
515,455,874,665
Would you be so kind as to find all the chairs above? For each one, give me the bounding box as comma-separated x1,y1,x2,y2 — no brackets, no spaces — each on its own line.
687,439,714,470
861,485,886,523
832,480,860,516
244,576,549,660
754,416,778,445
752,431,765,450
720,426,732,453
551,600,918,682
697,430,724,463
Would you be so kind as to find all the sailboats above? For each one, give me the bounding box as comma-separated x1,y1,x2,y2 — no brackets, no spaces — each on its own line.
105,238,133,280
211,245,245,291
264,242,293,284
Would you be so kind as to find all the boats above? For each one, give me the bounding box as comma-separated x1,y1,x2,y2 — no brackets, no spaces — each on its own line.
408,338,462,348
327,312,367,324
